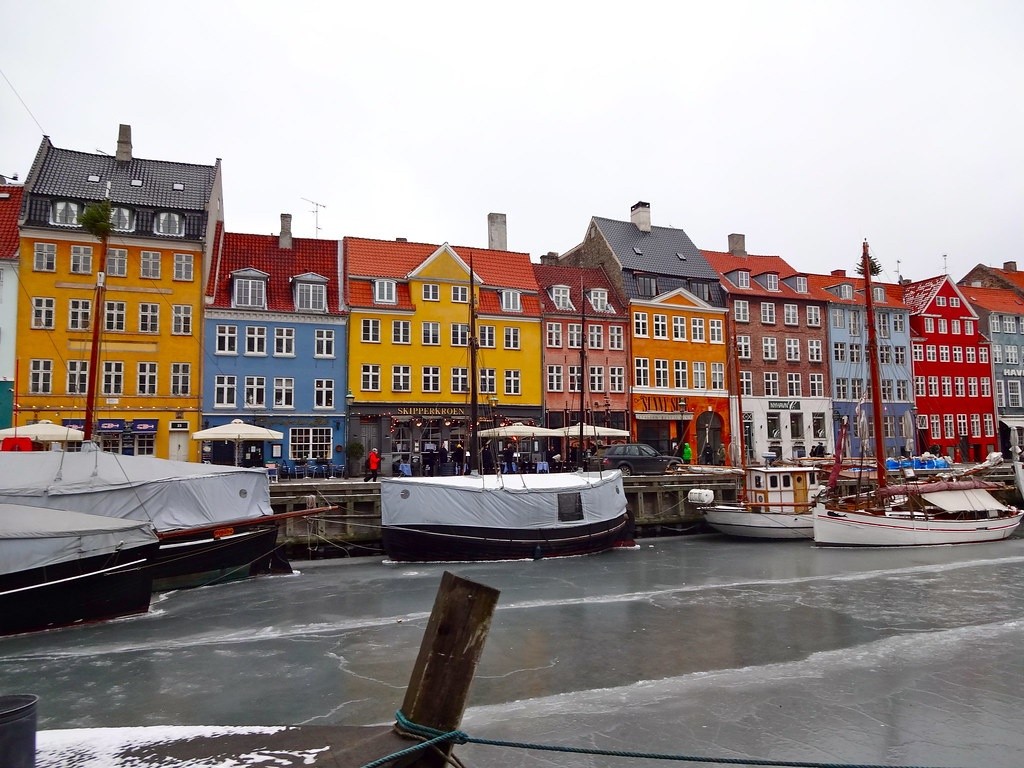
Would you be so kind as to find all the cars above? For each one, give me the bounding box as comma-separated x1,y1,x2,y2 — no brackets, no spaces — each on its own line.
587,443,685,477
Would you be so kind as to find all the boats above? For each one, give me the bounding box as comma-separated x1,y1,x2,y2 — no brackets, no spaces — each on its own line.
0,498,338,635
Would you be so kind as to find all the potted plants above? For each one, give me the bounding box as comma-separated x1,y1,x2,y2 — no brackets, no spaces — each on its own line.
346,440,365,476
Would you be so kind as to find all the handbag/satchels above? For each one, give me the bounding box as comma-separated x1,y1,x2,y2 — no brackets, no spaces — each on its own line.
464,465,472,475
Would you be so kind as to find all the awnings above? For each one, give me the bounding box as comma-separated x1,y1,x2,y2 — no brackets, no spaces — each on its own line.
999,418,1024,428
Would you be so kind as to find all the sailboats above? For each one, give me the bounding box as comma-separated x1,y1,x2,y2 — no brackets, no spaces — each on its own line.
809,236,1024,547
688,316,909,541
380,249,633,562
0,233,294,592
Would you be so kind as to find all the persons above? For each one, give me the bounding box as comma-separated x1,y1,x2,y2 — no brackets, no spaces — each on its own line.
717,444,727,466
671,446,680,457
364,448,383,481
703,443,714,465
815,443,825,457
454,444,466,475
503,443,515,474
482,444,492,474
682,443,692,464
439,443,448,476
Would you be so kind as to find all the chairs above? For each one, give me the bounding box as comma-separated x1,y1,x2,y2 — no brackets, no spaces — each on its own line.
395,461,575,477
268,462,344,482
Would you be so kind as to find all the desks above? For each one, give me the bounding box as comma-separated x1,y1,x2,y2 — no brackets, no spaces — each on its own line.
537,461,550,474
500,462,516,473
399,463,412,477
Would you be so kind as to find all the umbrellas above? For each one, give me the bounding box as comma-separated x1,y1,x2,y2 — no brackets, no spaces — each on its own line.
0,420,101,451
465,422,567,461
554,422,630,457
905,409,914,458
859,410,871,458
1009,427,1022,463
190,419,283,467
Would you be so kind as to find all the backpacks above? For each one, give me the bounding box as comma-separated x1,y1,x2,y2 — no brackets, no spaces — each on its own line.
365,458,370,469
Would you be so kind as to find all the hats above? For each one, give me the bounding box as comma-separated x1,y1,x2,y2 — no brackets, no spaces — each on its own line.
457,444,461,448
372,448,377,452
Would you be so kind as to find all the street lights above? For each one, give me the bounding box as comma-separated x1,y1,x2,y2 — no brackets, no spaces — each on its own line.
910,404,921,457
488,395,500,427
344,386,356,479
677,397,688,450
